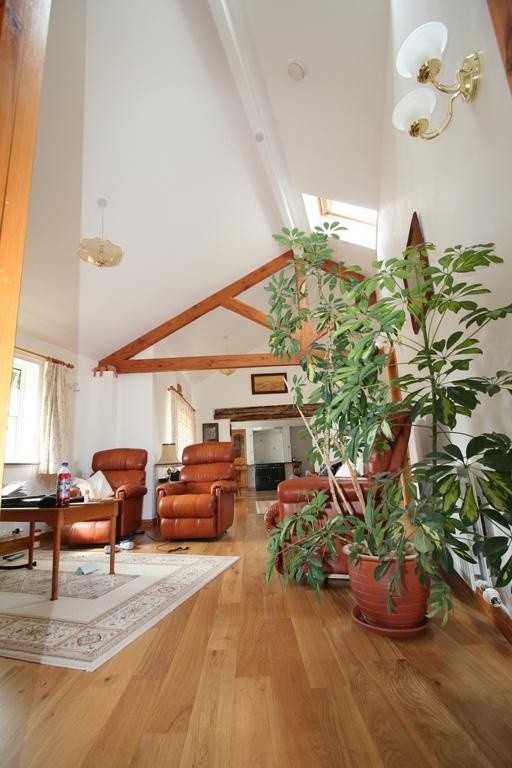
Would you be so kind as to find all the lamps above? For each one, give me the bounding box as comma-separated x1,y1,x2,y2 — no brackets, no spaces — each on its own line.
155,443,184,483
391,20,484,142
77,199,124,267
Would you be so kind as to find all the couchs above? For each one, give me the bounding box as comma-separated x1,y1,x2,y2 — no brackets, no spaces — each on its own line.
155,440,236,540
264,406,412,588
55,448,148,546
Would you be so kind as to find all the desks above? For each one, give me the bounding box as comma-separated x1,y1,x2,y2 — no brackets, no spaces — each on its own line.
0,496,122,601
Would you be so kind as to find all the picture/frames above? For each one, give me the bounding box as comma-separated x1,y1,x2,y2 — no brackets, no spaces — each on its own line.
202,423,218,443
251,373,288,395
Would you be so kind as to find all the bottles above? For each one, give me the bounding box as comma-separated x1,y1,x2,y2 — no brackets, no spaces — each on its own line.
56,461,72,507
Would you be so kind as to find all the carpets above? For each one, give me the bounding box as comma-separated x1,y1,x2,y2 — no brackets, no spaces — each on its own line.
0,546,241,673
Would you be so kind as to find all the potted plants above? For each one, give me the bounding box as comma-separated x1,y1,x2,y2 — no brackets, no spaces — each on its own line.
262,219,512,639
